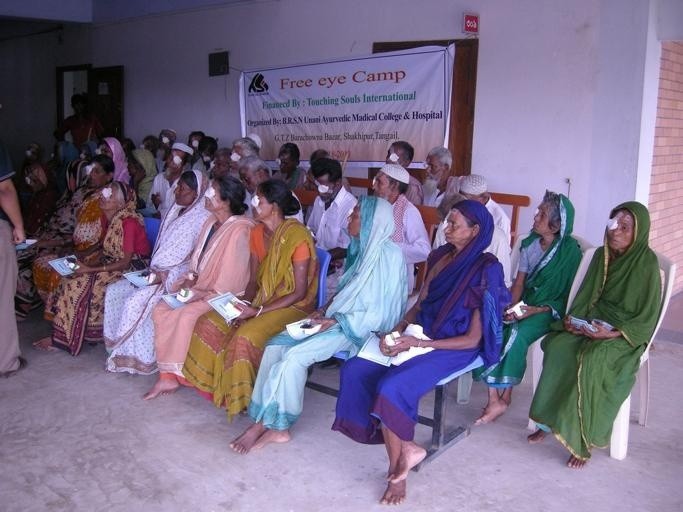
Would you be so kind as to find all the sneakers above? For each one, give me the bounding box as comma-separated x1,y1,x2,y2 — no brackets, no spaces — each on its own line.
0,356,28,378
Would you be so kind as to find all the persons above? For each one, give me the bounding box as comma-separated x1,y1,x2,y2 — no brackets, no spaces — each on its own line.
474,188,583,428
17,127,511,378
0,137,29,380
55,94,106,149
330,199,513,506
524,200,660,470
142,175,256,402
182,179,320,423
228,195,409,453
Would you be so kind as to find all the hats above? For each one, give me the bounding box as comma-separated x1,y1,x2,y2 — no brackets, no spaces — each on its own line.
171,143,194,155
379,163,410,185
246,132,262,149
460,173,487,196
